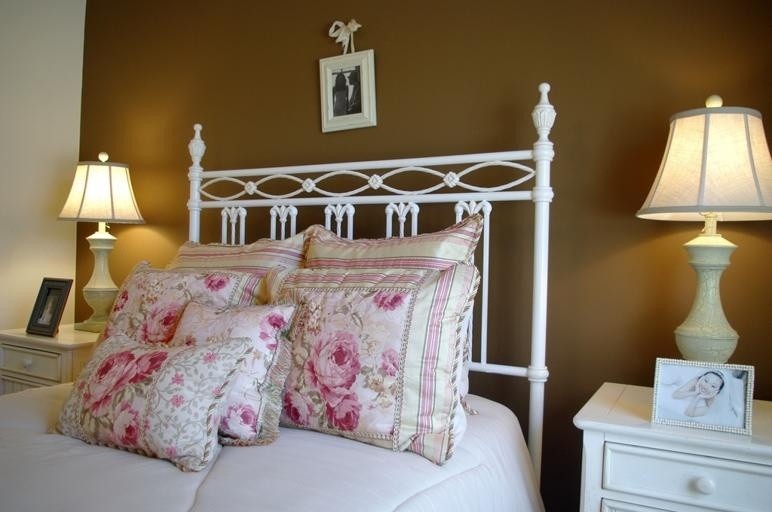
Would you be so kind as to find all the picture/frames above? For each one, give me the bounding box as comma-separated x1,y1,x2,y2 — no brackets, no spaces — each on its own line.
25,276,75,339
650,354,755,438
319,48,377,135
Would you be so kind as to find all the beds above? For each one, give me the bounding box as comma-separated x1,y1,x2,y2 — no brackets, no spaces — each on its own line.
0,78,560,512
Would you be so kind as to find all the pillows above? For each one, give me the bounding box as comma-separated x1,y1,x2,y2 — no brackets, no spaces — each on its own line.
116,273,254,343
76,337,218,462
187,309,292,439
278,271,465,451
310,232,483,269
169,234,301,273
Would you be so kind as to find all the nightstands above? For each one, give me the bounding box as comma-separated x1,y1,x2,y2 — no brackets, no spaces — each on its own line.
571,378,772,512
1,321,103,395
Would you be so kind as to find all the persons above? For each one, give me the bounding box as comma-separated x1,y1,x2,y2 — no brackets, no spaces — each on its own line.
331,73,350,116
347,70,362,113
671,368,727,422
38,297,54,326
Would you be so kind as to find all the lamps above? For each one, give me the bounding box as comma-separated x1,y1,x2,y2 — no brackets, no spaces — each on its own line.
55,148,147,335
634,95,771,364
